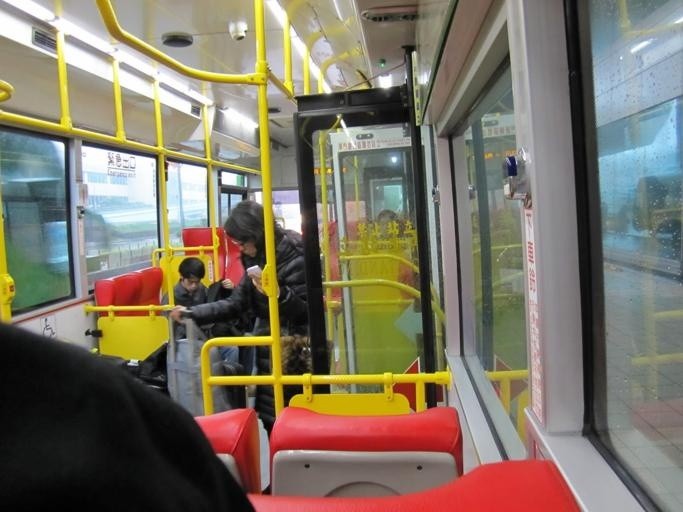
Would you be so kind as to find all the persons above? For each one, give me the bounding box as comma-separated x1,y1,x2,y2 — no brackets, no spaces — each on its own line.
373,210,412,260
162,257,241,375
169,198,312,440
0,320,256,511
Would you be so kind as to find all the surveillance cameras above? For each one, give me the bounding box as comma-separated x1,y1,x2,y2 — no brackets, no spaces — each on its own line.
228,22,248,41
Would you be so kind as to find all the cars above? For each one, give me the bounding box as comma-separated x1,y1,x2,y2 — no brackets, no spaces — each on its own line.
607,175,682,250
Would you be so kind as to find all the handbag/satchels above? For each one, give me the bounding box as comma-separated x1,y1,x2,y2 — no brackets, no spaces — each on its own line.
166,317,231,417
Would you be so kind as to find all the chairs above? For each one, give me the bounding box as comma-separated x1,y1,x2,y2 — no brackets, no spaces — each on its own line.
93,226,247,316
194,407,262,494
269,405,464,497
321,219,417,315
246,458,585,512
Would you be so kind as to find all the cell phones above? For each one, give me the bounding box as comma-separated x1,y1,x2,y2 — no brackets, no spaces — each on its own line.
246,265,262,278
